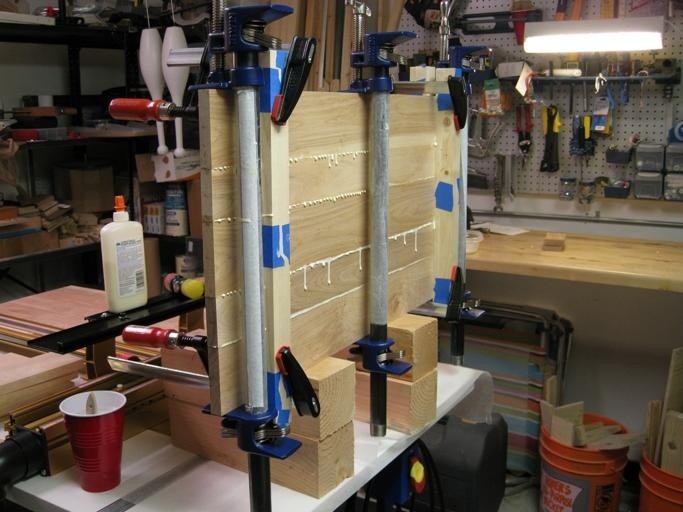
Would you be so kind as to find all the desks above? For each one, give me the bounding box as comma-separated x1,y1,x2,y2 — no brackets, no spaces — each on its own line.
0,362,494,512
466,229,683,293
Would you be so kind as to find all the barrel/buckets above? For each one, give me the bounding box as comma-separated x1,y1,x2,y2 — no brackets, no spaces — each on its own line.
637,446,683,512
539,414,631,511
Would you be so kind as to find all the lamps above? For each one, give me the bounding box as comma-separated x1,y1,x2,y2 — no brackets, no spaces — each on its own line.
522,15,666,53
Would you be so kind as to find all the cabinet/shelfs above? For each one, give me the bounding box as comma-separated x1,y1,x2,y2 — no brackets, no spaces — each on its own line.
0,0,162,294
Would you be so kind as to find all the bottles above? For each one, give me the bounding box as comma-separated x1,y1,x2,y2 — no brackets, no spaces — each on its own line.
58,389,128,494
559,176,595,206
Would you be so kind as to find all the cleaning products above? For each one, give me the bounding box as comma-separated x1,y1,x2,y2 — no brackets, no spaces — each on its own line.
100,194,149,314
